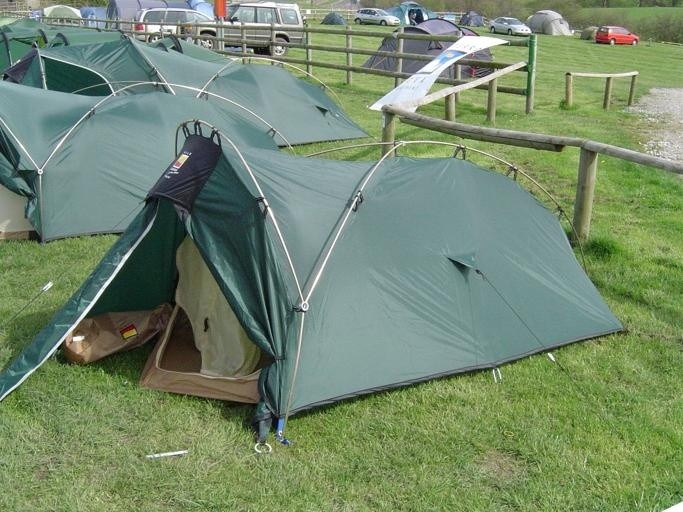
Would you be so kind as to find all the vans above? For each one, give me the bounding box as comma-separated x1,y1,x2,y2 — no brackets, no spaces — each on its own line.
133,7,214,44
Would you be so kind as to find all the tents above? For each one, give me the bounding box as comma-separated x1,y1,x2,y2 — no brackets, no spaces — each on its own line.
458,11,485,29
392,2,435,27
526,11,573,37
1,119,626,444
1,2,373,245
360,19,494,81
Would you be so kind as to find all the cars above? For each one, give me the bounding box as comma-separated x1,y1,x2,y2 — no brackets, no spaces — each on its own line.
486,16,532,36
595,25,639,45
353,8,401,26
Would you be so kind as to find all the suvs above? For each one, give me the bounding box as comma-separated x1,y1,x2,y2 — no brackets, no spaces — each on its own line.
179,2,303,58
